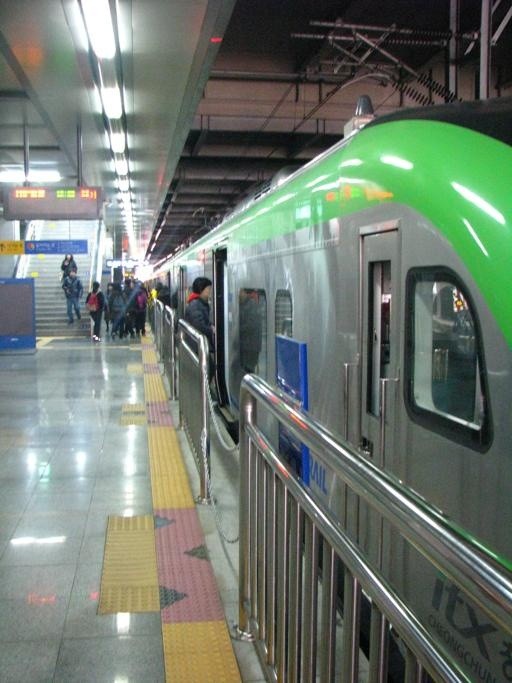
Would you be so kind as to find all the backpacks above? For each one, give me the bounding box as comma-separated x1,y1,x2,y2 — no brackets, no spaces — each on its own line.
87,293,98,312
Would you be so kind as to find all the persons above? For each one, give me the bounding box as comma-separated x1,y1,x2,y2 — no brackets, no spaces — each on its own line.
62,268,83,322
86,279,170,341
61,254,77,284
184,278,216,382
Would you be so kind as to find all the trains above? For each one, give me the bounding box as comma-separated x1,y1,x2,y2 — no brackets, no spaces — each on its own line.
140,97,511,682
